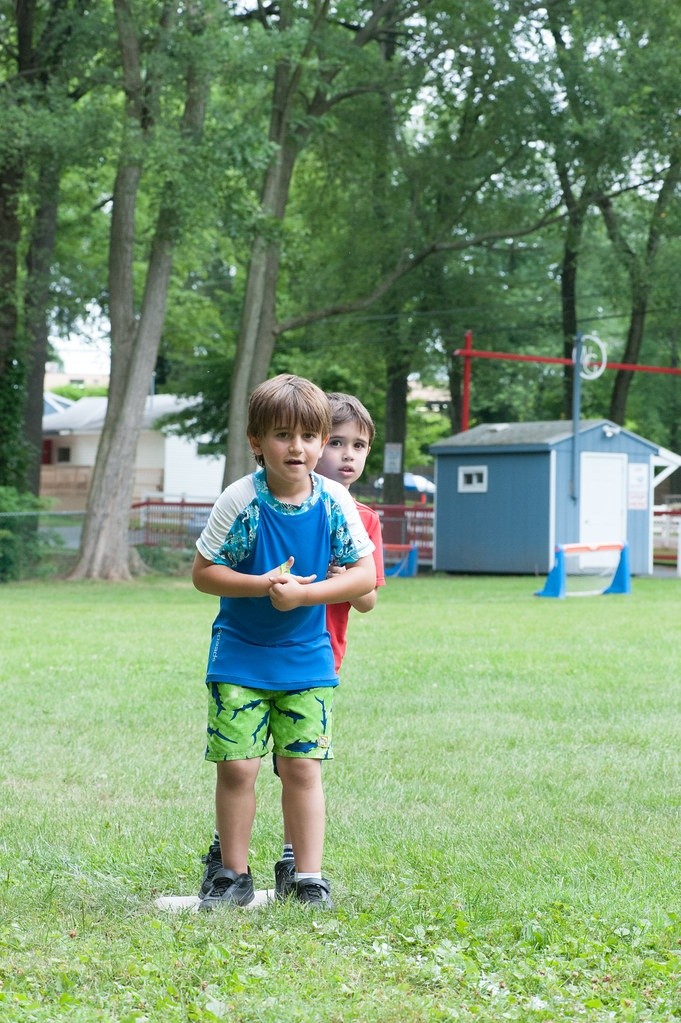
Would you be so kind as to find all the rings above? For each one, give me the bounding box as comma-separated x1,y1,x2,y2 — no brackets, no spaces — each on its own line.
280,562,290,575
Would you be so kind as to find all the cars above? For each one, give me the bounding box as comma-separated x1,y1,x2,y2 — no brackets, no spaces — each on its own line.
373,469,434,502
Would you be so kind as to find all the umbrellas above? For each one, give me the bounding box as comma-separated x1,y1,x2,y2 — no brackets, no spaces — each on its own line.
374,471,436,493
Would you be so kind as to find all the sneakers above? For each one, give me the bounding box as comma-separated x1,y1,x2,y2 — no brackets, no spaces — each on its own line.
275,858,295,907
296,877,338,913
198,844,224,900
198,865,254,916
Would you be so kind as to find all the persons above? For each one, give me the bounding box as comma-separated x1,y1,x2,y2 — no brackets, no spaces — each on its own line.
193,372,385,912
